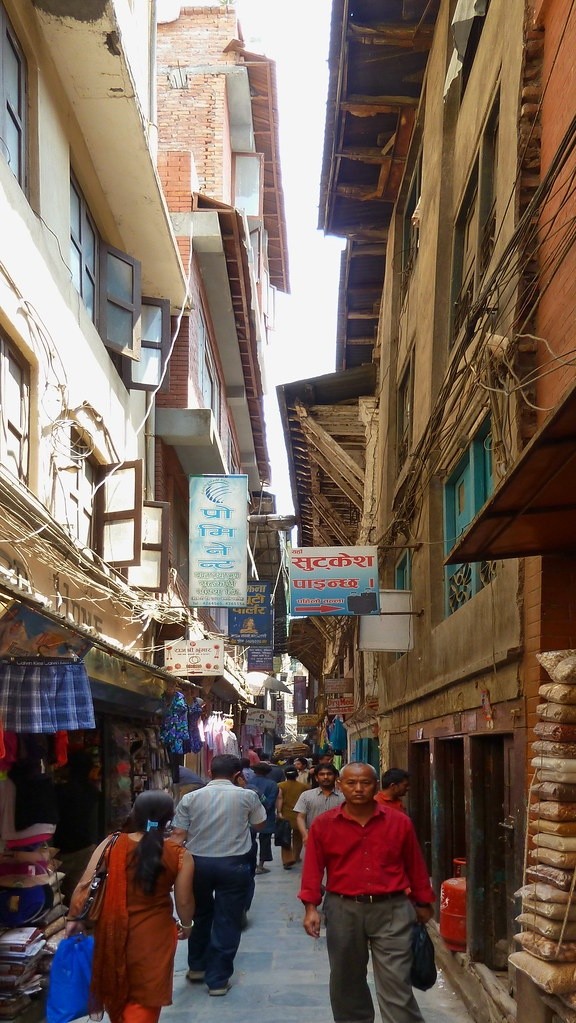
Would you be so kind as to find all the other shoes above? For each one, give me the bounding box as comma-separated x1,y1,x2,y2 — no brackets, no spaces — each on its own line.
296,859,304,863
208,980,233,996
186,970,206,982
283,864,292,870
255,865,271,875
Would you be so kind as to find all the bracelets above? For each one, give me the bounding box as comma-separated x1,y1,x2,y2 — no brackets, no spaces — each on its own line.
178,919,194,931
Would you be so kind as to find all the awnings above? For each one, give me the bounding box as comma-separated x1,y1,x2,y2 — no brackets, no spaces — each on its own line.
0,584,205,691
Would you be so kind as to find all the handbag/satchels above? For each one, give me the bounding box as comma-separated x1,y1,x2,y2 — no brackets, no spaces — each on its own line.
46,932,94,1023
410,923,437,992
274,819,292,847
67,831,121,921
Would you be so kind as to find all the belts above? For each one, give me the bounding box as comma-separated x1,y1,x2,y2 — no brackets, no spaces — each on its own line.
335,890,404,904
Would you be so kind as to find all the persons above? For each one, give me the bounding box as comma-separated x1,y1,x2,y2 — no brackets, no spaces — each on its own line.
170,754,267,996
64,790,195,1023
240,617,259,637
296,761,437,1023
228,745,410,873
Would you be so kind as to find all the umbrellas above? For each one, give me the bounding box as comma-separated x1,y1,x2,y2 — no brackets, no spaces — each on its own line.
245,671,292,704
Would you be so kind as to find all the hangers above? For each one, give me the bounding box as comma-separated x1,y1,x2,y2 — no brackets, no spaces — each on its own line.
211,711,230,732
